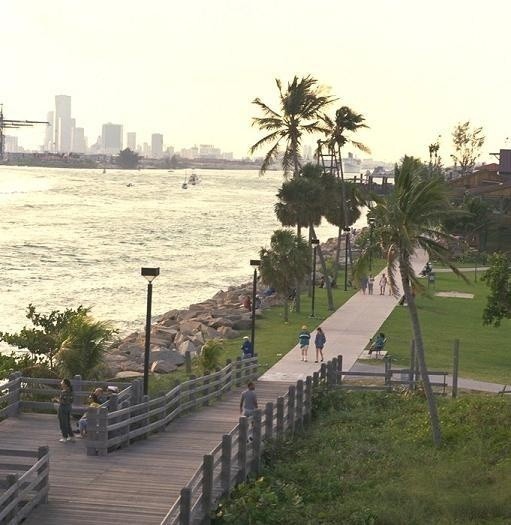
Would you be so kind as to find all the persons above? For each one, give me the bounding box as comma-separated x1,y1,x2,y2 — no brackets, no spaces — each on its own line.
241,335,255,376
312,327,326,363
239,262,434,312
71,387,108,433
365,332,387,357
239,383,260,446
105,385,119,399
73,394,102,439
53,378,75,443
298,324,311,362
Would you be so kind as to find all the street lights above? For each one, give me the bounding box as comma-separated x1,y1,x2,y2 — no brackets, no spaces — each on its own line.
248,259,262,355
369,217,376,273
140,266,162,396
343,226,351,290
311,239,320,318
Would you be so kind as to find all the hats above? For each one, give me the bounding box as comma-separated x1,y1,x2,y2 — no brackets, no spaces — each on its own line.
242,336,249,339
302,326,307,329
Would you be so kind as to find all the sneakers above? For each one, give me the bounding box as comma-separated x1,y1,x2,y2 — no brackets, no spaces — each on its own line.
59,438,67,442
67,437,73,441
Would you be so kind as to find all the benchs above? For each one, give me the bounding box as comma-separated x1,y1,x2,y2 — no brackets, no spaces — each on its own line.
370,336,391,359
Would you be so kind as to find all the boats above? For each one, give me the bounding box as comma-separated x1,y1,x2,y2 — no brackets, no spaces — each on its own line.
182,181,188,189
188,170,202,185
